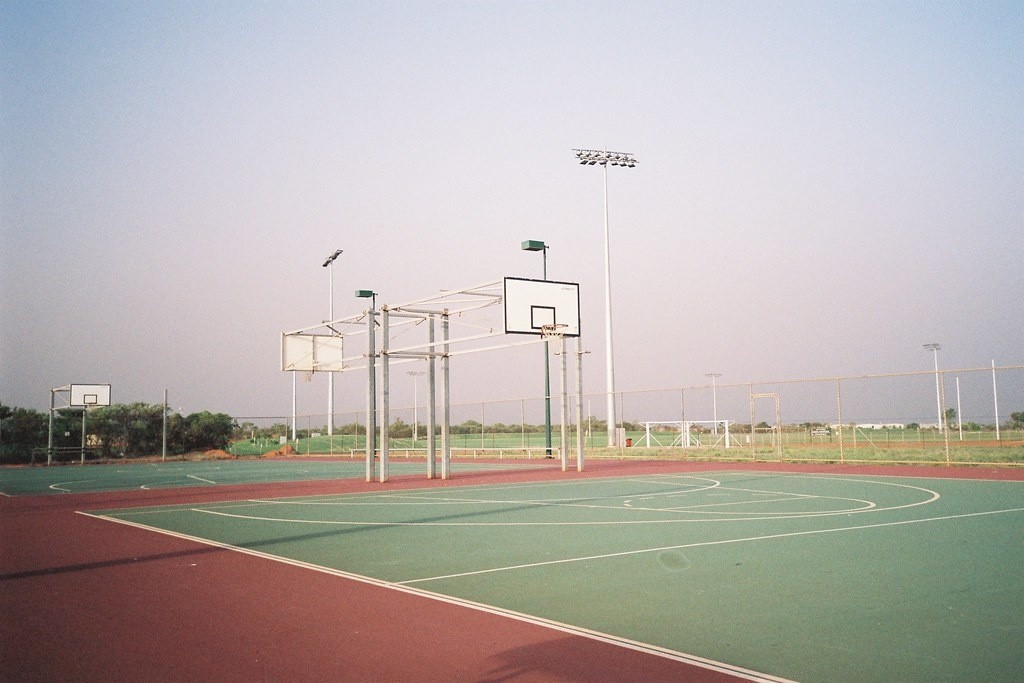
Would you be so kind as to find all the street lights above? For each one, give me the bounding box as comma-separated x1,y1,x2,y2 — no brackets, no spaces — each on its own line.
704,373,724,432
571,148,640,446
923,343,941,437
323,249,344,435
355,290,377,457
521,240,552,460
179,406,188,463
